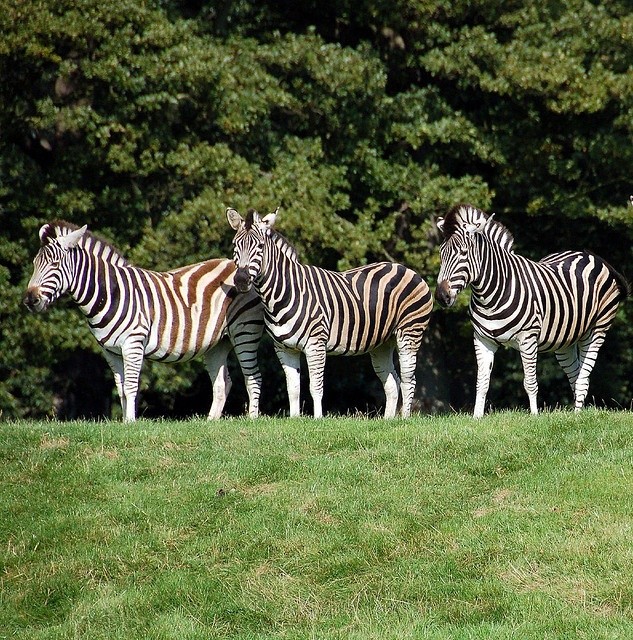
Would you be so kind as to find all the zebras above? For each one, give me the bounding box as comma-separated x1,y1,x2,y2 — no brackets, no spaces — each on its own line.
434,205,632,420
225,206,434,420
23,219,265,423
